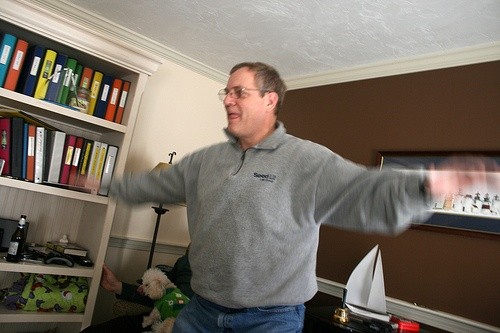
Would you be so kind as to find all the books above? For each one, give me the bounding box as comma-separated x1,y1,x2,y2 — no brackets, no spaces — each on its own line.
0,34,131,197
47,241,88,256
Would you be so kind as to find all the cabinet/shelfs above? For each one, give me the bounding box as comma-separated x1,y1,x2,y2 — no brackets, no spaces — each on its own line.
0,0,166,333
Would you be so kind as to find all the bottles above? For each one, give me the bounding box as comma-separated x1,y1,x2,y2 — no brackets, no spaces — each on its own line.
7,215,27,262
436,189,500,215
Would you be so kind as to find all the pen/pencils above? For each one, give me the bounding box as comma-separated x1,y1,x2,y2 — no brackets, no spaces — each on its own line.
343,289,347,309
334,323,360,333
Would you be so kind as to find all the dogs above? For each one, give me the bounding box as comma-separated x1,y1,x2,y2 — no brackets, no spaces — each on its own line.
136,267,192,333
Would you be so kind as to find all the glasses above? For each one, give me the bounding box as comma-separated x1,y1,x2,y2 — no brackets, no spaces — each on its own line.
216,87,277,101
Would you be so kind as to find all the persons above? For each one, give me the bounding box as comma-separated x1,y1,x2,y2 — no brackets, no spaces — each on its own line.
85,62,500,333
81,243,194,333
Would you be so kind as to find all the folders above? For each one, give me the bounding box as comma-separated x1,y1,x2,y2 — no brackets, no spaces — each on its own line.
0,111,119,197
0,33,131,124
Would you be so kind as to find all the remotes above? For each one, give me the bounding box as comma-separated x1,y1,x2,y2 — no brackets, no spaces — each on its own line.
73,255,94,267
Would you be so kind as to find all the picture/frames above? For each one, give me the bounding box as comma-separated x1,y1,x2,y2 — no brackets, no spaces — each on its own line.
369,147,500,241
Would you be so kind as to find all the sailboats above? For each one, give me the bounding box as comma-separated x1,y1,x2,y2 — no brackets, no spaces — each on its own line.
341,244,393,323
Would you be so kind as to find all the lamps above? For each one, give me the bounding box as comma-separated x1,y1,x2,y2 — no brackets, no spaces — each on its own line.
136,151,188,285
